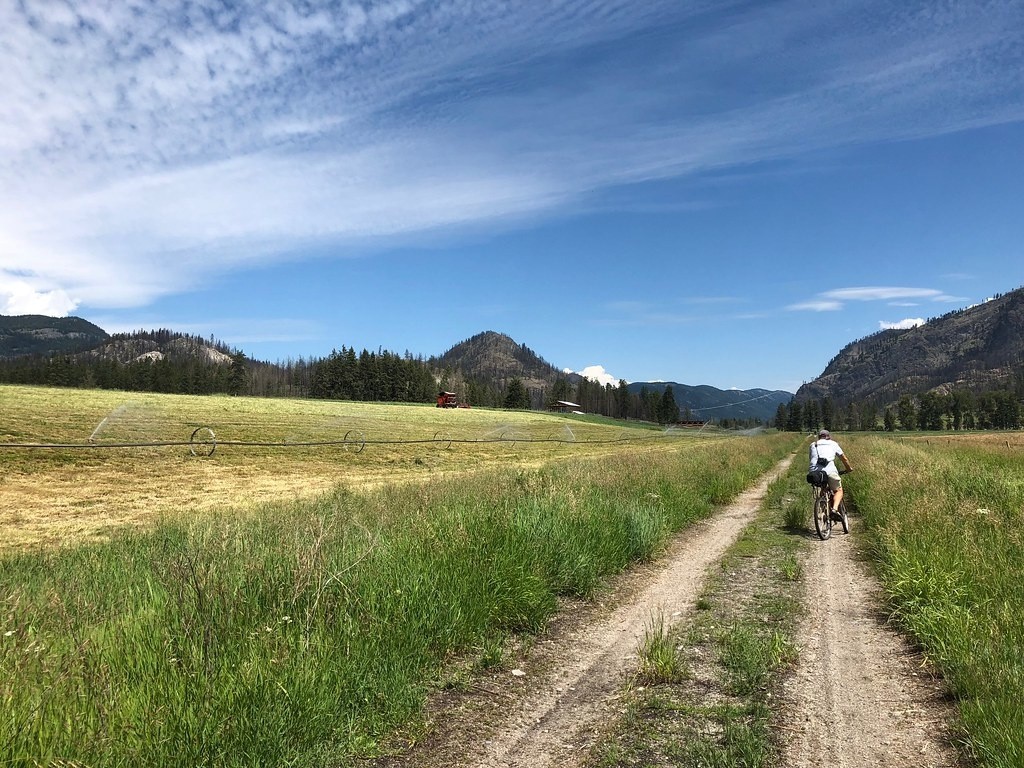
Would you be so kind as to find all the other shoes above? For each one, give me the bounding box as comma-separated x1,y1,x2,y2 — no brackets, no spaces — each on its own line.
830,510,843,522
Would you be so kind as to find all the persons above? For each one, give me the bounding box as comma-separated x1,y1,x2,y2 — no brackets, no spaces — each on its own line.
808,429,852,521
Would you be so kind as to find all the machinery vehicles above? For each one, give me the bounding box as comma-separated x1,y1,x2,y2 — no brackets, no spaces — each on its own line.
436,392,471,408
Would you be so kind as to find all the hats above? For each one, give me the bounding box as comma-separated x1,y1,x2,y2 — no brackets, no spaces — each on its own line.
819,430,829,439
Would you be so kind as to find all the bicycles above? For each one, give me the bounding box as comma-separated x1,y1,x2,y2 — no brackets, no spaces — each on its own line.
813,469,852,540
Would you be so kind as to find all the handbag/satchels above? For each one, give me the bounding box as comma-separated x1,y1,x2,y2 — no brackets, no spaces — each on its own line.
807,471,827,487
